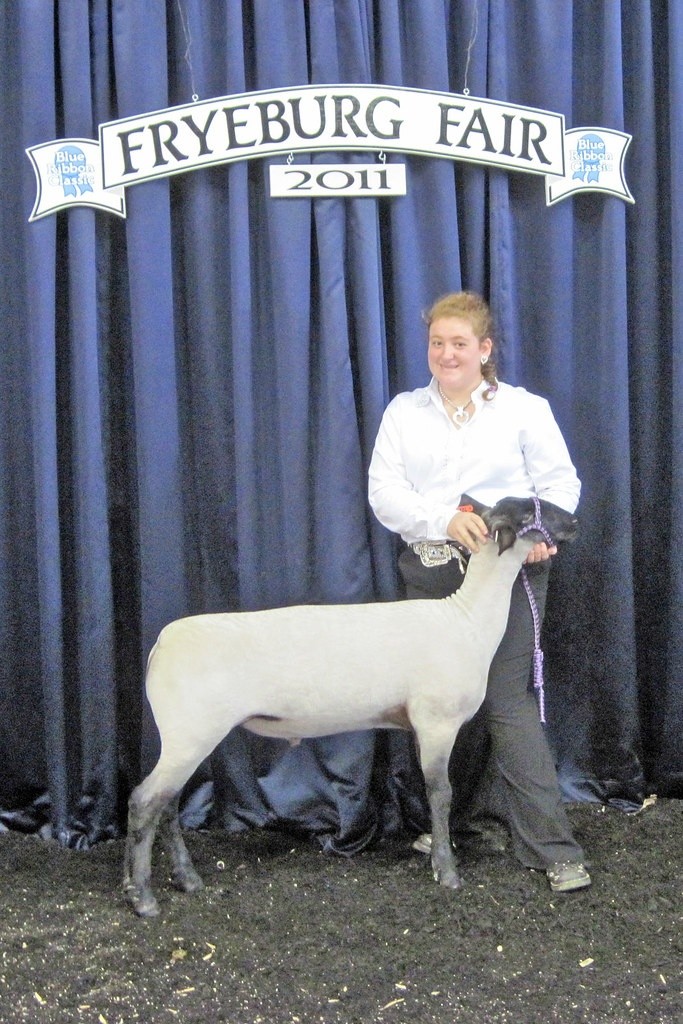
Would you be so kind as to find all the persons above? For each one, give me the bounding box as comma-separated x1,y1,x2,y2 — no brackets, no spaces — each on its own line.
368,292,592,891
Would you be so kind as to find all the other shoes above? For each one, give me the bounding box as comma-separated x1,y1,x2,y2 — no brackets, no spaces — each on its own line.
411,830,465,856
546,860,591,892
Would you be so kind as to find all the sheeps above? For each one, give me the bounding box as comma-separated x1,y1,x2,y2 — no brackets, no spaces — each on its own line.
123,495,583,918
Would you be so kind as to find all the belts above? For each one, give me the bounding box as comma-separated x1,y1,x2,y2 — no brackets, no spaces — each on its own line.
413,541,471,574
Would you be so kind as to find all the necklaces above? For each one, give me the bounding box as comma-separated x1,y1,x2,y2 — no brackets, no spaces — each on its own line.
439,386,472,427
